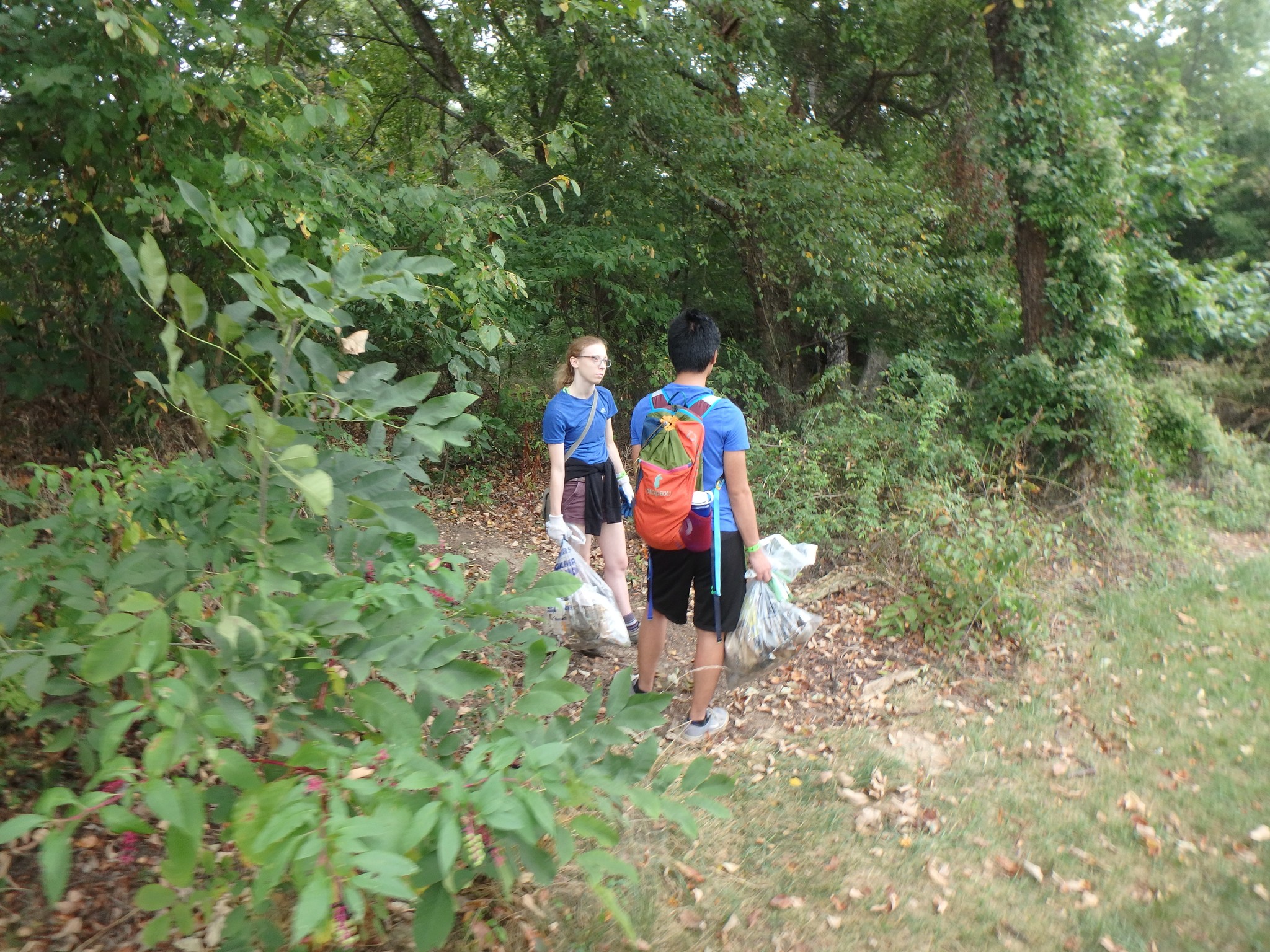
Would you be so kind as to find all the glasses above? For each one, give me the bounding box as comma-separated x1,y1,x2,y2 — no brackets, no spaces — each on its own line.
573,355,612,368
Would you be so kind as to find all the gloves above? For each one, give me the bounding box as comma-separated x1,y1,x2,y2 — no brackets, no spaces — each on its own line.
615,471,635,518
547,514,568,547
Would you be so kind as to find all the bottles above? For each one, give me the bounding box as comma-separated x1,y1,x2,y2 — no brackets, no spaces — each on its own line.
690,490,714,517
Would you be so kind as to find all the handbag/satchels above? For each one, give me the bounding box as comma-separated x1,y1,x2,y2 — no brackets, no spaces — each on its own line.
541,487,550,523
542,523,633,652
723,533,824,691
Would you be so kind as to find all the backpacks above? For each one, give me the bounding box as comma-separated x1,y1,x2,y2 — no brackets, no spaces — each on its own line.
631,389,722,553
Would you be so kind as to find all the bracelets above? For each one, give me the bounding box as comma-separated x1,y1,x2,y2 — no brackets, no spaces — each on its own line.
744,540,762,552
616,473,628,480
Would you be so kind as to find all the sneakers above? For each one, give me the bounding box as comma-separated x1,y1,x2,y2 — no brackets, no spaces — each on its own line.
682,706,729,742
627,621,641,647
580,648,601,657
613,670,640,695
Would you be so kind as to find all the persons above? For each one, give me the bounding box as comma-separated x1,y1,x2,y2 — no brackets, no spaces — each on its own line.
624,308,772,741
542,336,641,656
